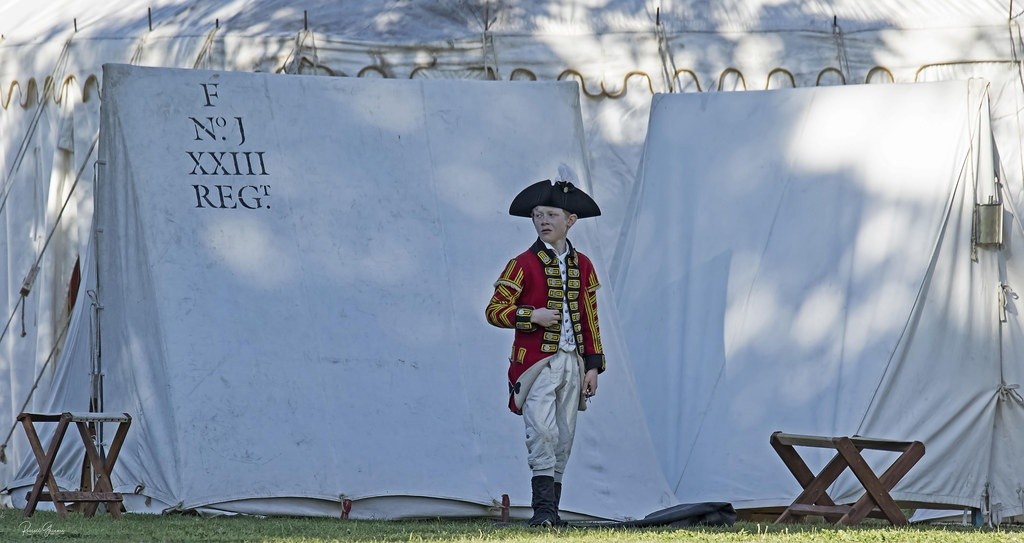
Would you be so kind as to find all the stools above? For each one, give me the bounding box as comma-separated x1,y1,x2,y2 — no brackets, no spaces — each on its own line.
770,430,926,526
18,411,131,516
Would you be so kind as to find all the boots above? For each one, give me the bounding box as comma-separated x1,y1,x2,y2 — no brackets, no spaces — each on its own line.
528,481,562,525
528,475,556,528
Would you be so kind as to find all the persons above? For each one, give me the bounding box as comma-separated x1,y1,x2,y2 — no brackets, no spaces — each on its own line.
485,179,606,528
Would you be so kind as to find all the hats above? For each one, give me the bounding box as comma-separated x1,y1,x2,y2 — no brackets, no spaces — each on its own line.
509,179,601,219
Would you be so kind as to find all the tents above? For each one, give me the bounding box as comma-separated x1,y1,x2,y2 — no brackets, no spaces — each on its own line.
0,0,1024,510
